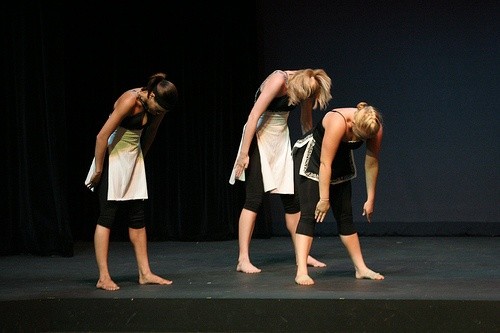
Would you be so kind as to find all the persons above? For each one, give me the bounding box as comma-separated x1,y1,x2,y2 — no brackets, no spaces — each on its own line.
291,102,386,286
84,73,177,291
228,68,332,273
